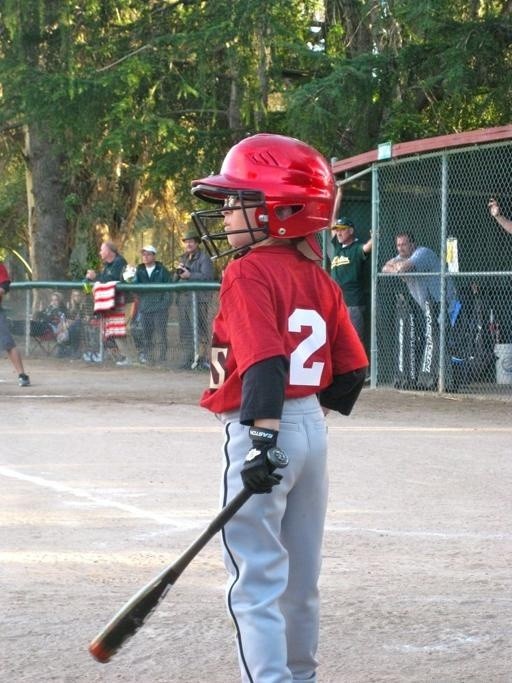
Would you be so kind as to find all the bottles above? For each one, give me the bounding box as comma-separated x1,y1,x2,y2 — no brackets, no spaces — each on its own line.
486,306,495,342
494,322,500,344
82,277,92,295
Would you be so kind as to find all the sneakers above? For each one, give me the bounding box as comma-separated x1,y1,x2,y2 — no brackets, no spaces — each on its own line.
116,354,133,366
19,374,29,385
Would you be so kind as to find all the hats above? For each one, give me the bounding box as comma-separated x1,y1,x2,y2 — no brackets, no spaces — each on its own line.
182,232,201,244
140,245,157,255
332,217,353,230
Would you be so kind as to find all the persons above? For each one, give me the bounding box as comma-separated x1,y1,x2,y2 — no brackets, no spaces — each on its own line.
34,238,171,369
329,186,372,351
187,134,370,682
487,198,512,235
172,229,220,371
381,231,460,392
0,260,29,388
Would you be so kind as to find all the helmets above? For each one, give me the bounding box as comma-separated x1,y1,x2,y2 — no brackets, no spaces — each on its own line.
190,133,337,262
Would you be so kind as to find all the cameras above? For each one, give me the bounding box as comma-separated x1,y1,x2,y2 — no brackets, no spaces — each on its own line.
176,265,190,274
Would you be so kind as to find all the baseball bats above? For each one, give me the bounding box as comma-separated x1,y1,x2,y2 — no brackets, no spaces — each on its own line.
88,447,290,663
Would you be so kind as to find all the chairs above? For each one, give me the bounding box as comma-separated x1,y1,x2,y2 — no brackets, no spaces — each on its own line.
29,328,53,355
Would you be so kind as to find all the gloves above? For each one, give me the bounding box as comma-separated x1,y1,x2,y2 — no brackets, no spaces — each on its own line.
240,427,283,494
